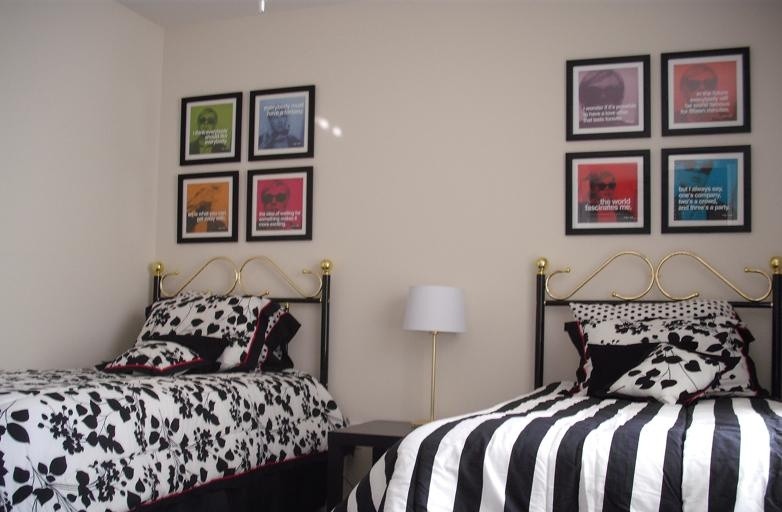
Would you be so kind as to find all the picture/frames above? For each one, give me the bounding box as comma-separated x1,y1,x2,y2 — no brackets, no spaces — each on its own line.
565,148,651,235
246,166,313,240
176,170,239,243
566,53,651,141
248,85,315,161
660,45,750,136
661,144,752,233
179,91,242,166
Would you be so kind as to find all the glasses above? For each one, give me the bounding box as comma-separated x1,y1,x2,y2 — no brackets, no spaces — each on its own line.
264,194,285,202
598,183,616,189
199,117,215,124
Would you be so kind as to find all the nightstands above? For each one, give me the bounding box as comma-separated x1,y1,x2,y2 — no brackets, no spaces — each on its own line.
324,419,422,512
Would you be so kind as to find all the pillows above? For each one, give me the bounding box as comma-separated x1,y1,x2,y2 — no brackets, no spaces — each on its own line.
562,300,769,407
95,292,301,378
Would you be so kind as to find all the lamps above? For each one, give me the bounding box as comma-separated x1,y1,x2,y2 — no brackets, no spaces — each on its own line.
401,284,467,429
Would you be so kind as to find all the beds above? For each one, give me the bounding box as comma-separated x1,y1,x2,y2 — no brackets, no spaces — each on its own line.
323,251,782,512
1,255,332,512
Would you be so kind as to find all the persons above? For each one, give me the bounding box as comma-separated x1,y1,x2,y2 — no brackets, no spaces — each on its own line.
258,105,304,149
674,159,737,220
256,181,300,230
674,64,736,123
578,172,637,222
579,69,635,128
189,107,231,154
186,185,228,233
